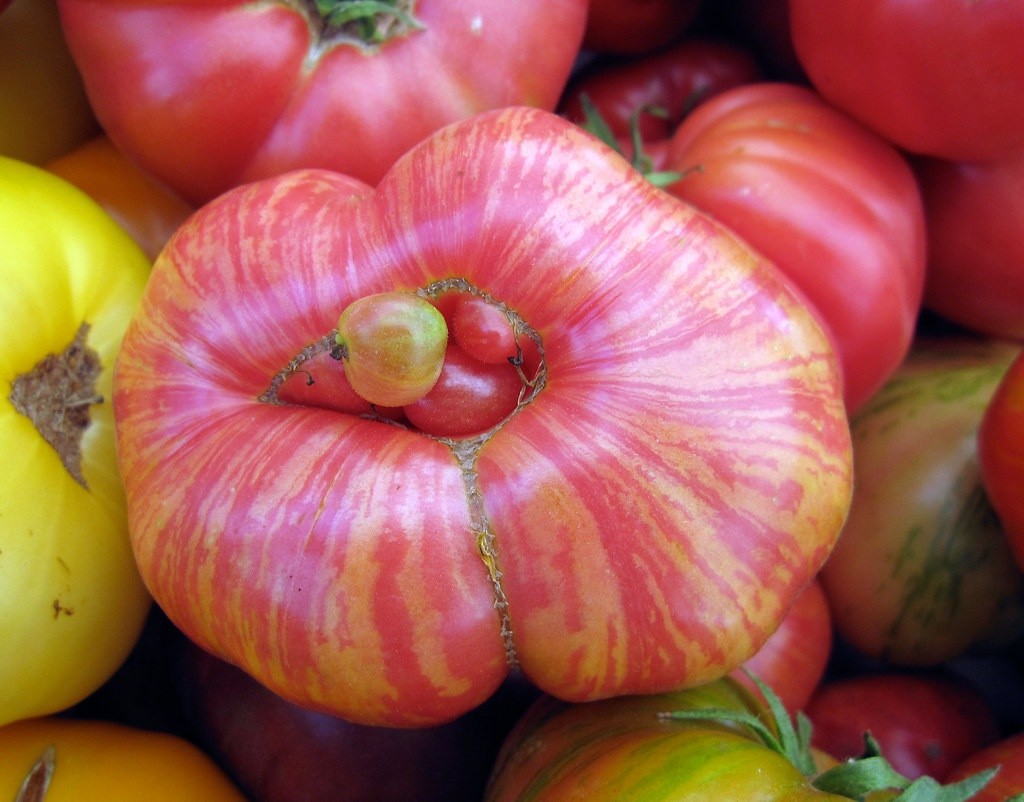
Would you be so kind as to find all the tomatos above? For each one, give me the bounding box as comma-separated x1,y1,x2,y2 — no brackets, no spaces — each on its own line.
0,0,1024,802
